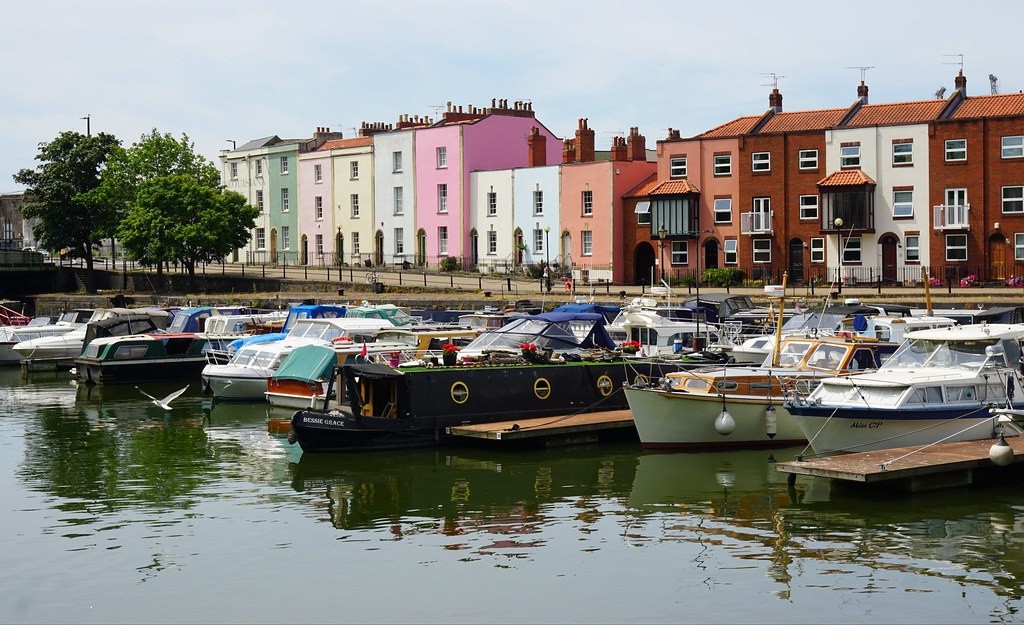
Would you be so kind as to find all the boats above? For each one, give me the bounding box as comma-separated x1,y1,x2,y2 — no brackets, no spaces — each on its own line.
0,292,1016,450
780,323,1024,460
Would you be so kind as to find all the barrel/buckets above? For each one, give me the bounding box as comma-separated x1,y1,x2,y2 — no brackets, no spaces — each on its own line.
237,323,244,332
674,338,682,353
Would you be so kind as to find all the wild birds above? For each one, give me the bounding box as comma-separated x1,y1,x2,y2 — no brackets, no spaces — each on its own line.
135,384,191,410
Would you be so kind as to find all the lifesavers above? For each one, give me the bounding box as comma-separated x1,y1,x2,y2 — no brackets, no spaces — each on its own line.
564,282,572,292
331,336,352,345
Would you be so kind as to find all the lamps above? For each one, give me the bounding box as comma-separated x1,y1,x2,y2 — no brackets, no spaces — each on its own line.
994,223,999,228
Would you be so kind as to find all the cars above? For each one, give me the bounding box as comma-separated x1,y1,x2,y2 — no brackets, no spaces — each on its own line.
22,246,50,257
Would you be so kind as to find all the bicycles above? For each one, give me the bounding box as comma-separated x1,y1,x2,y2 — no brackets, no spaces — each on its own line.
366,270,377,284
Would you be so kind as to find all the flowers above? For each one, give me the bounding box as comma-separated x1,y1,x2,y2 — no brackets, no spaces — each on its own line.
388,351,401,358
620,340,643,354
441,343,460,356
520,343,537,354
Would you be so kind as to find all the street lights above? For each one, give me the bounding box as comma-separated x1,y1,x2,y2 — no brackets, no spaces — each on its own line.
544,225,551,292
658,226,668,287
336,224,342,281
834,218,844,294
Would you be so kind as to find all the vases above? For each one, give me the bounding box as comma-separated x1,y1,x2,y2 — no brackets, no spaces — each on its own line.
443,355,456,366
523,353,535,363
388,358,399,368
623,349,635,356
